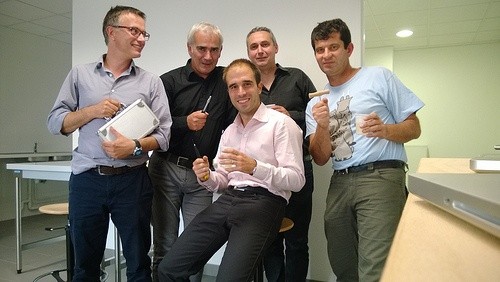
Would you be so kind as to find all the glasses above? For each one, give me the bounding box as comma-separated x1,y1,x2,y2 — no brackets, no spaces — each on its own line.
112,26,151,41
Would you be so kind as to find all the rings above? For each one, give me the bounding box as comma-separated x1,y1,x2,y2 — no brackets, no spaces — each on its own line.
232,159,234,164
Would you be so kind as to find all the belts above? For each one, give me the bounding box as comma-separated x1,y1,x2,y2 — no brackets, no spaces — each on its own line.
161,151,194,170
334,160,404,175
230,185,278,198
95,163,146,175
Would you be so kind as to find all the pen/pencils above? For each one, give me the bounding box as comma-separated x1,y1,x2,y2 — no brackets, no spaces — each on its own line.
193,142,209,180
202,96,212,113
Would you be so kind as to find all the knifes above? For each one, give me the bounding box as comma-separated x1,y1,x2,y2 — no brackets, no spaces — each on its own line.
193,142,209,181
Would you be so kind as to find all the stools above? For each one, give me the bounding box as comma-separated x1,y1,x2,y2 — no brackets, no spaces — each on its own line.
32,202,109,282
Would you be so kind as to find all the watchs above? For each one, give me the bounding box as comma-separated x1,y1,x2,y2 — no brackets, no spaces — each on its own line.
133,138,142,157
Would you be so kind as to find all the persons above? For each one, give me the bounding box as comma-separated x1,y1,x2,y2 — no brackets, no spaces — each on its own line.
246,26,317,282
306,19,425,282
157,61,306,282
159,23,238,282
48,5,172,282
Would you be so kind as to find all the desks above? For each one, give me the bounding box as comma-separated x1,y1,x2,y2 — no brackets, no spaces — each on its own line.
6,160,126,282
374,159,500,282
